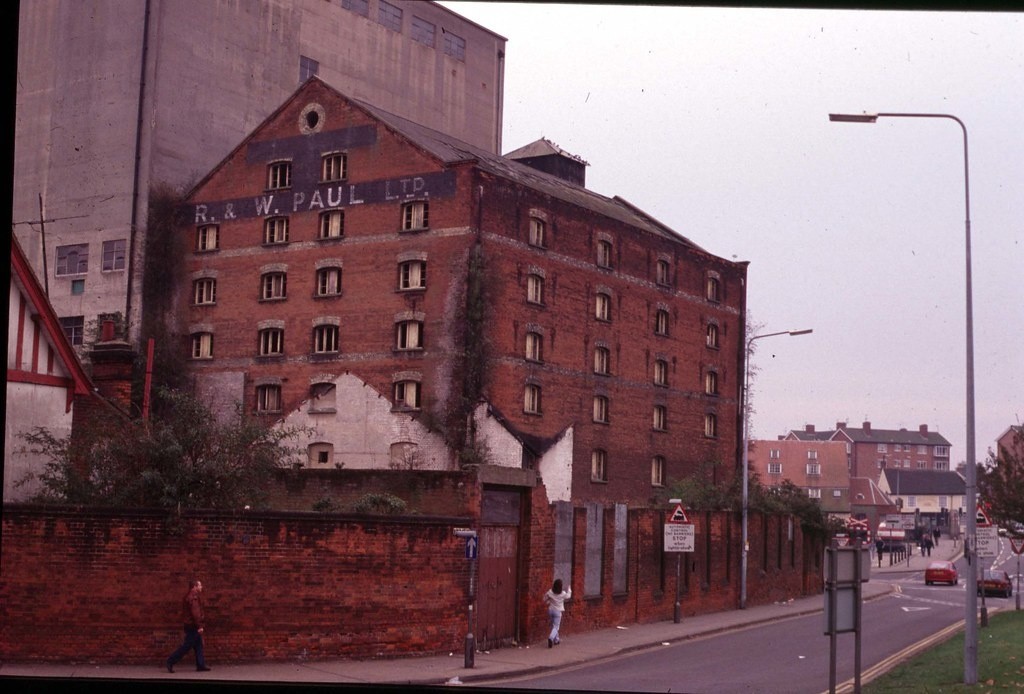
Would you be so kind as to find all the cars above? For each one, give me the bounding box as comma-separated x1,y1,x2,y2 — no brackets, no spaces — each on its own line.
924,561,958,586
977,569,1013,598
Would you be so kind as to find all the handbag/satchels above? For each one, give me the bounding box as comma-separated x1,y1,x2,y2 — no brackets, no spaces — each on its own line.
546,594,551,607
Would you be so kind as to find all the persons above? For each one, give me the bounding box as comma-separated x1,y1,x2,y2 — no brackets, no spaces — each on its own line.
868,524,943,561
542,579,573,648
165,578,213,673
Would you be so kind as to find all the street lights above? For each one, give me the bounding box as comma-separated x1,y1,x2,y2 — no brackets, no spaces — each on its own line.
830,110,982,688
737,328,814,609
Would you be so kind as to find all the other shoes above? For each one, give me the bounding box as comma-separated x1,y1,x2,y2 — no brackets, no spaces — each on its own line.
197,667,211,672
548,639,553,648
166,660,175,673
554,640,560,644
